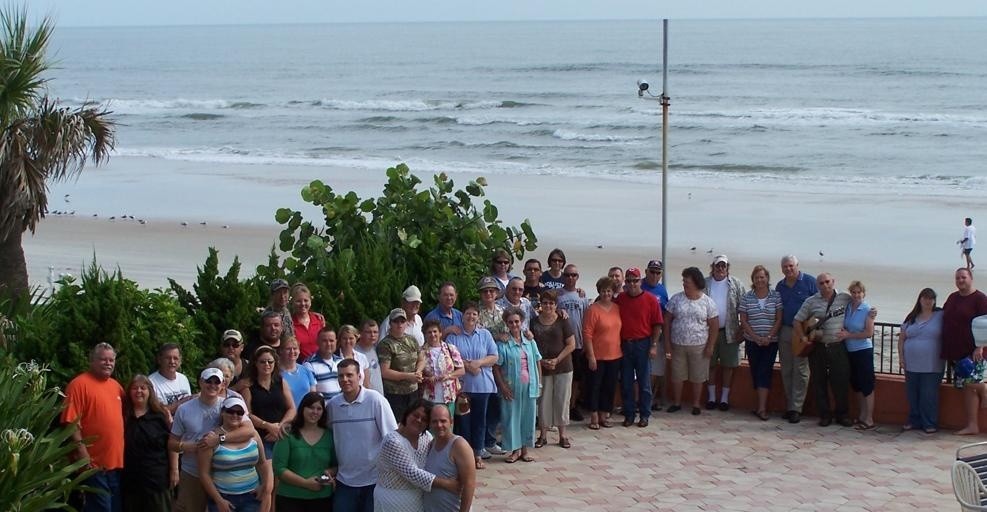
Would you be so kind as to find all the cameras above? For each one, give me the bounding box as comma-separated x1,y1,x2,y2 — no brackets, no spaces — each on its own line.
317,474,331,484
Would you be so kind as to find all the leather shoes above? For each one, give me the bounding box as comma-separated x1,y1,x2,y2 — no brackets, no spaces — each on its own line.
750,409,879,431
637,419,648,428
622,419,633,427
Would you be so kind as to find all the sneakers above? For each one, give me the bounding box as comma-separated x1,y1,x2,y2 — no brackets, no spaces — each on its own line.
602,418,614,428
588,400,730,416
569,409,584,421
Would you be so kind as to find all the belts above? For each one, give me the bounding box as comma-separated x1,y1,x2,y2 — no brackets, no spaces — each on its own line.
622,337,650,344
816,341,842,348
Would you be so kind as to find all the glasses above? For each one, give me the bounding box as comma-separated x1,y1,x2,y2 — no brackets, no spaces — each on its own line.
551,258,564,264
647,270,663,275
494,260,509,265
563,272,577,277
523,268,540,273
624,278,641,283
301,391,326,399
198,327,278,418
817,280,830,285
504,320,521,324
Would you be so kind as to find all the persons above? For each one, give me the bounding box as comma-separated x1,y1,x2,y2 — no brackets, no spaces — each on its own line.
424,249,667,467
898,268,986,435
961,218,977,269
664,255,877,430
61,278,476,512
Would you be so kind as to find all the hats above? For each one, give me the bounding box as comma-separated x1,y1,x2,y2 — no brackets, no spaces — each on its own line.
954,359,977,377
649,259,663,270
712,254,729,265
389,308,408,321
624,267,641,277
400,285,422,303
476,277,501,293
270,279,289,291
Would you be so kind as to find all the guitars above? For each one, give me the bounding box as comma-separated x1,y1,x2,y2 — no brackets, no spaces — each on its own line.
793,306,845,357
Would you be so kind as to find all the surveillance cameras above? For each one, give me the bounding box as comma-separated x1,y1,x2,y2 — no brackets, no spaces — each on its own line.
637,78,649,97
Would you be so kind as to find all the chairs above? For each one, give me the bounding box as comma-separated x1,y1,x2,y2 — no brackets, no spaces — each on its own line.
952,461,987,512
956,442,987,490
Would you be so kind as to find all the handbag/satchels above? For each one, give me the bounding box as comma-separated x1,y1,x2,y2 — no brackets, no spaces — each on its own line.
455,393,471,415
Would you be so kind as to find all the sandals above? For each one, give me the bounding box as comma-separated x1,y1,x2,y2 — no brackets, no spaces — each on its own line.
473,436,571,470
589,418,600,429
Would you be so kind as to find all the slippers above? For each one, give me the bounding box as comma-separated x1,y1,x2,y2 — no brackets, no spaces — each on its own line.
902,423,918,430
923,427,936,433
